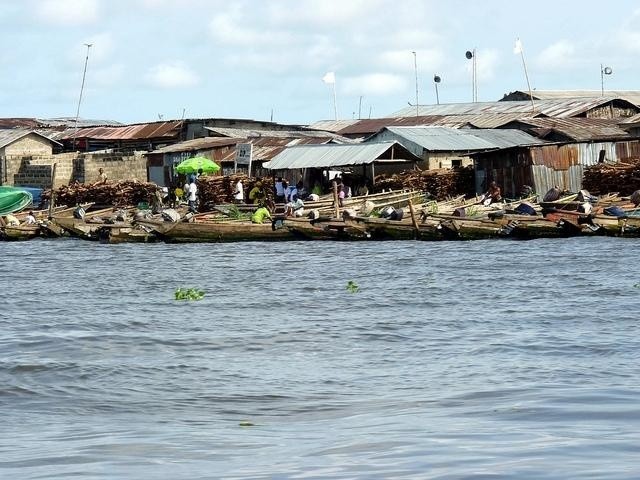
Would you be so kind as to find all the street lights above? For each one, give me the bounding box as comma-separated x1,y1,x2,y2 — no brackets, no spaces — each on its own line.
465,46,478,103
433,73,442,103
409,49,419,124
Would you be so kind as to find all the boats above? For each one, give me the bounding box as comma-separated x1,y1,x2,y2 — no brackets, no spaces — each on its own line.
0,187,640,244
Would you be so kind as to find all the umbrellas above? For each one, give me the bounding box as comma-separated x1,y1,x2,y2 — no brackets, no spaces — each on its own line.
175,156,220,173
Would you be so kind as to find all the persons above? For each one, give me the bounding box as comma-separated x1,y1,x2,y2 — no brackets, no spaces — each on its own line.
40,217,53,224
98,168,108,180
481,180,501,204
160,169,370,224
25,212,36,224
